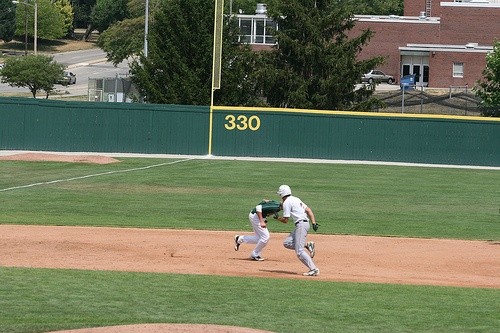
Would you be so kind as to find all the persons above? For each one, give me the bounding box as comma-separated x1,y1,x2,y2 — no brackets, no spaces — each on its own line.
234,184,320,276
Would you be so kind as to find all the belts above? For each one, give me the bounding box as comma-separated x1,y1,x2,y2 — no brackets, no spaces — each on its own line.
295,220,308,225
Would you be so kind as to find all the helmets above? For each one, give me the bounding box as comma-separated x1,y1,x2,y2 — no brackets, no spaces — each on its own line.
279,184,291,197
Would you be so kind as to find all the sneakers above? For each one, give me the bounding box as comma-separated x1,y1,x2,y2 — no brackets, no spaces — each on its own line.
303,269,319,276
234,235,240,251
308,241,315,258
250,255,264,261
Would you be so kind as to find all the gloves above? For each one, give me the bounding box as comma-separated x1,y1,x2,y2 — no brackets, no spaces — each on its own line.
312,223,319,230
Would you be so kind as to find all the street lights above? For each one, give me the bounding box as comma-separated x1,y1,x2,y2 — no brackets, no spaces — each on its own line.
12,1,38,56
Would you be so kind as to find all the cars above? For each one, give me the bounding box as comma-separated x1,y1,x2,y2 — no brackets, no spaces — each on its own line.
56,71,76,85
361,70,395,84
